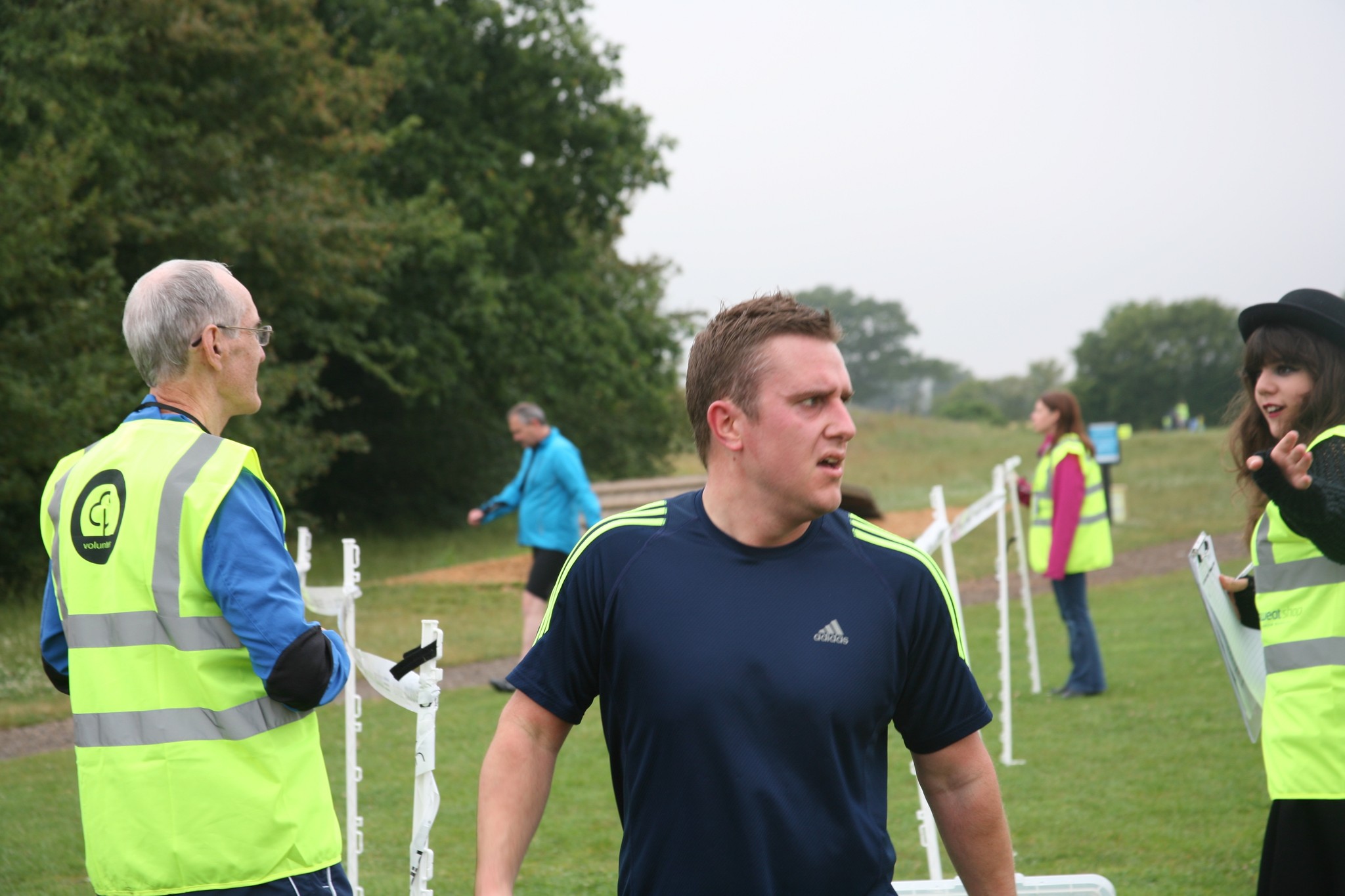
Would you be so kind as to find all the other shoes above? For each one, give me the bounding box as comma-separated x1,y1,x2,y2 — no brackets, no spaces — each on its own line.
490,679,516,692
1054,689,1087,699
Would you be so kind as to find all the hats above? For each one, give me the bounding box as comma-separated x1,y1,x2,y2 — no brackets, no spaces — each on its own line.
1238,289,1344,346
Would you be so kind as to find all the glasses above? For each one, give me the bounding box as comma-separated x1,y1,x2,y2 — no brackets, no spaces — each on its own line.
191,324,273,347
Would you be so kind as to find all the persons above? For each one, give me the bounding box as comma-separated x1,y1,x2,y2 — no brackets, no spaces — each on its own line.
466,288,1344,896
1011,393,1114,698
1218,288,1344,896
467,401,601,693
39,261,351,896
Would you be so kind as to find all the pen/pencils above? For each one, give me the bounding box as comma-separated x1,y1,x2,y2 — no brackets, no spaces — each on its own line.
1234,562,1254,579
